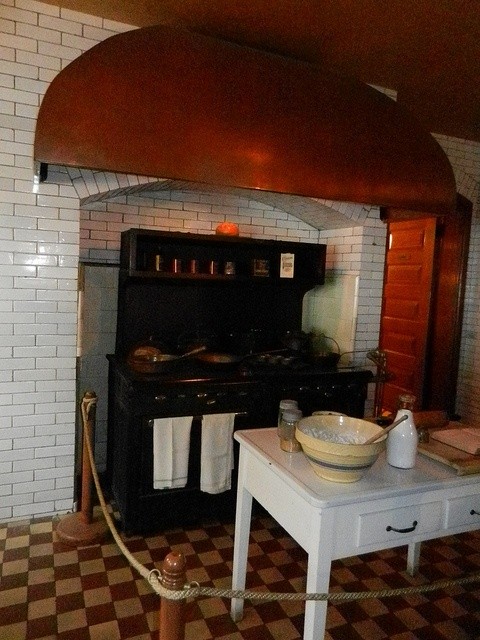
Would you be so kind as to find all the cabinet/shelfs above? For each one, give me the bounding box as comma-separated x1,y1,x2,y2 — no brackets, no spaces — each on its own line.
105,228,375,539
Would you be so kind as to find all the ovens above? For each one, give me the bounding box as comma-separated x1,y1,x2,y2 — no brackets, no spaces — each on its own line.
106,393,261,538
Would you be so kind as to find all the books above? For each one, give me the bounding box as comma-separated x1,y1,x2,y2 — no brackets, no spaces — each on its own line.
430,427,480,456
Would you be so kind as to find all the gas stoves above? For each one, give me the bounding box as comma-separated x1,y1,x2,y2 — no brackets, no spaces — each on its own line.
105,339,371,415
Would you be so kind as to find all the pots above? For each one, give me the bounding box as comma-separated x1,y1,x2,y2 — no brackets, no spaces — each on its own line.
130,347,206,374
304,352,341,367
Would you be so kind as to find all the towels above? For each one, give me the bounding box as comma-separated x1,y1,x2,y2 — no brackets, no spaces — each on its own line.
152,417,192,490
200,412,235,495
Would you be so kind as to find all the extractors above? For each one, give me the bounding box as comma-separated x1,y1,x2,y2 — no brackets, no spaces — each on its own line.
34,25,458,215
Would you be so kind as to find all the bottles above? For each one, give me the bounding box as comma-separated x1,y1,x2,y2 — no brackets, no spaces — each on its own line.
278,400,298,438
207,255,217,275
172,251,182,273
387,394,418,470
418,425,430,443
154,247,163,271
188,254,198,274
223,256,236,276
281,412,302,453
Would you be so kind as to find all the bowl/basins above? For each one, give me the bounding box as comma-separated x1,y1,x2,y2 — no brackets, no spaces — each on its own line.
295,415,388,483
193,352,252,375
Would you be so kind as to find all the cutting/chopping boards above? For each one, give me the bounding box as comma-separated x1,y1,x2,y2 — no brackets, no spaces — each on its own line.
417,421,480,476
